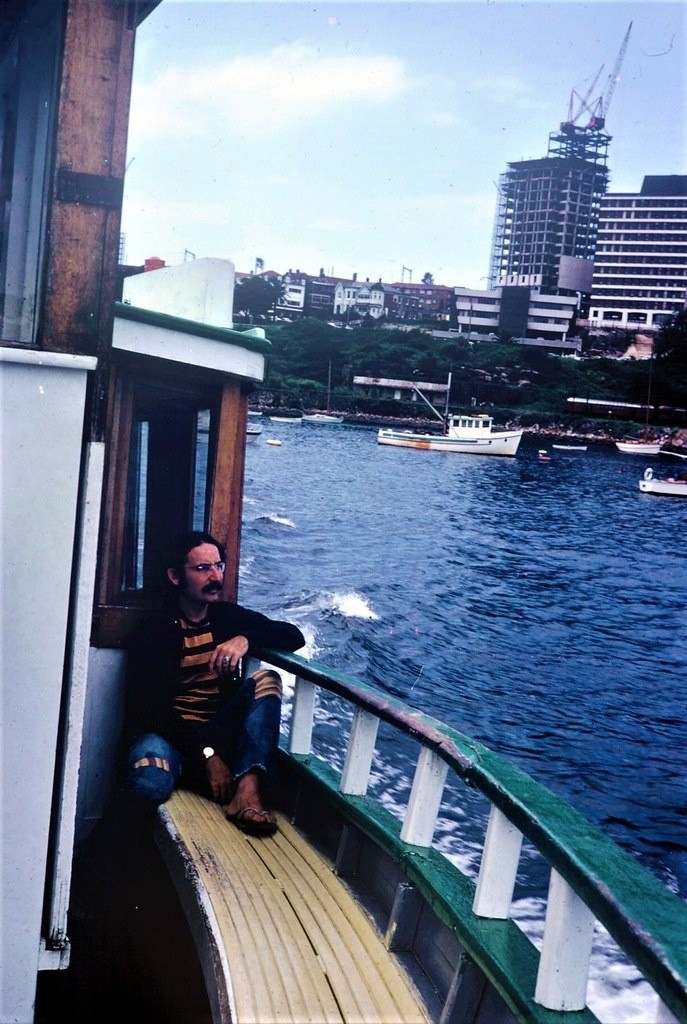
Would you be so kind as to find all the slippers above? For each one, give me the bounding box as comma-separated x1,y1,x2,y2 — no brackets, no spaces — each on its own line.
225,806,278,834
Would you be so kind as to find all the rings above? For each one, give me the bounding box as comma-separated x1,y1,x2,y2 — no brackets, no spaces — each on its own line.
222,655,230,664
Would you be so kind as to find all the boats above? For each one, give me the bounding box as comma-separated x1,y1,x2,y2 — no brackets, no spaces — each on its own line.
303,414,345,423
247,409,263,416
271,416,303,423
244,421,264,442
636,466,687,496
376,383,524,455
615,436,662,454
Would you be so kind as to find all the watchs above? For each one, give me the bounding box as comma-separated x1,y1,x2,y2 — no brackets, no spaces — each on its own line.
199,745,218,761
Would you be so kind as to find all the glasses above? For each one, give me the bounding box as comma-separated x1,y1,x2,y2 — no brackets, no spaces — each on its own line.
175,561,225,573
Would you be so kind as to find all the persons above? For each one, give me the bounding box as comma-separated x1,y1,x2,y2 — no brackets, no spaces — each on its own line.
97,528,306,908
